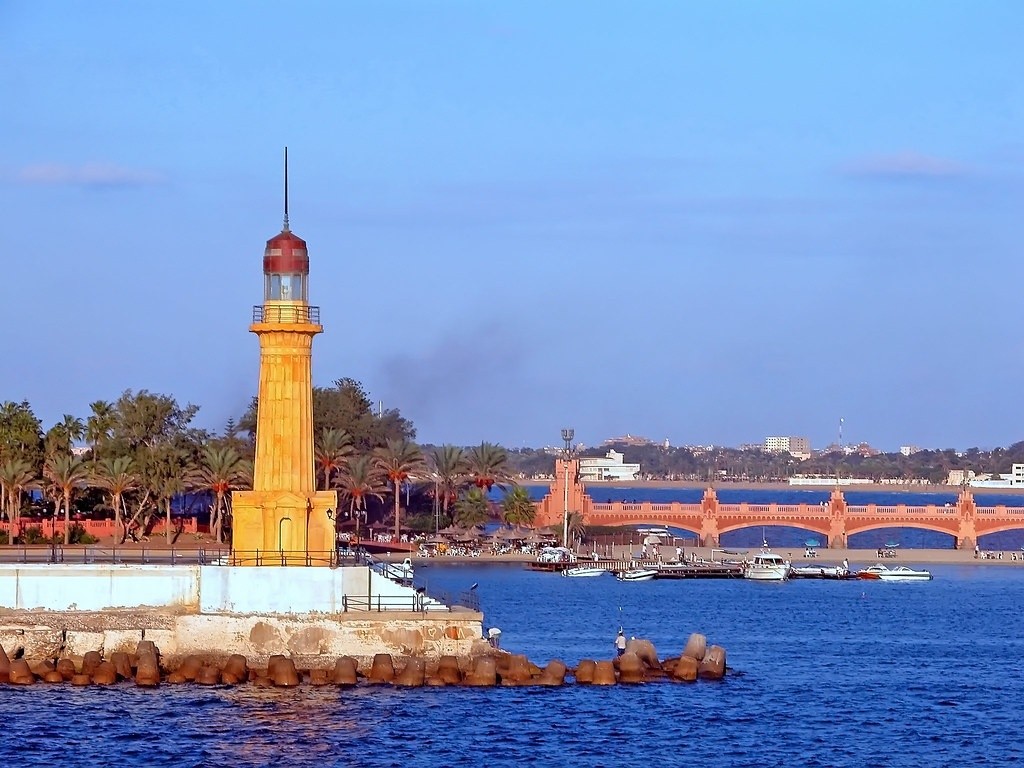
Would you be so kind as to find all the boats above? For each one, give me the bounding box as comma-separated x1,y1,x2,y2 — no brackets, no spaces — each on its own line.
636,528,671,537
789,564,837,575
804,538,821,547
369,557,414,586
857,566,890,573
878,567,930,581
885,540,899,547
561,567,606,577
743,554,791,580
616,569,658,581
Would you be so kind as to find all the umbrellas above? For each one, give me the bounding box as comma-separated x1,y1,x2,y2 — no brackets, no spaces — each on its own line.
805,540,820,546
425,524,485,543
482,526,554,544
885,540,899,548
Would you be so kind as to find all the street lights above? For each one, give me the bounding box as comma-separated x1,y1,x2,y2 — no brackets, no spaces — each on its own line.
563,464,569,547
51,505,56,561
326,508,349,565
220,506,233,555
353,508,367,563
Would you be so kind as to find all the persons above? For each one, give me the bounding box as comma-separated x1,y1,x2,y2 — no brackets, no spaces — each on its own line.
675,546,683,554
614,631,627,656
974,544,979,558
806,548,813,554
843,558,849,569
653,546,656,556
348,540,354,556
986,549,990,556
643,544,647,559
878,548,888,554
486,627,501,644
